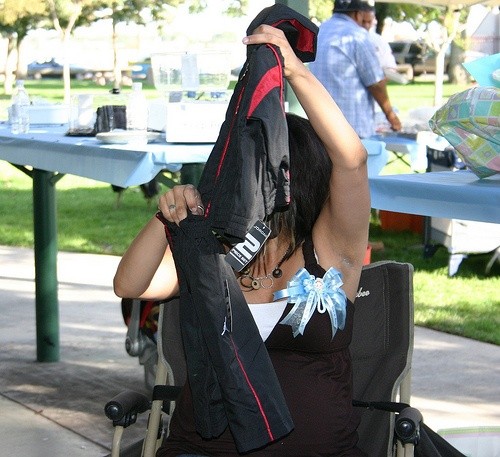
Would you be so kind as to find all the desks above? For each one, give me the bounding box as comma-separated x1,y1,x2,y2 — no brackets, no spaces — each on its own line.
0,120,218,364
367,170,500,225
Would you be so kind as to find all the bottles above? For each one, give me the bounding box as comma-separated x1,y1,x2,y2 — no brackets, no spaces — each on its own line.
125,82,149,147
11,79,30,133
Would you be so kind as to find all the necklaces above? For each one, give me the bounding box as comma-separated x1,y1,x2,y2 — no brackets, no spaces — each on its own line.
236,243,293,292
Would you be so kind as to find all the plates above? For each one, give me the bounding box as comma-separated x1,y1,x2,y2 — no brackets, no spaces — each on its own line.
97,131,128,143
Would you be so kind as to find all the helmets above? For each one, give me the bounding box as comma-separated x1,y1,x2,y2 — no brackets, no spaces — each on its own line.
333,0,376,11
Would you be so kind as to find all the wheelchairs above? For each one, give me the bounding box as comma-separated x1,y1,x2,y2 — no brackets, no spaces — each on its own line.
105,258,423,456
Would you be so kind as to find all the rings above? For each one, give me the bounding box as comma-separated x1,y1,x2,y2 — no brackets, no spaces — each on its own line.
198,205,204,215
168,204,175,210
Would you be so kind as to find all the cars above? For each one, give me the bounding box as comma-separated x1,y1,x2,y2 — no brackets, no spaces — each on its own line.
27,59,86,81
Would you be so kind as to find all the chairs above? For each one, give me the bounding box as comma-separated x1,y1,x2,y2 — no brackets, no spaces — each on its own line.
105,259,422,457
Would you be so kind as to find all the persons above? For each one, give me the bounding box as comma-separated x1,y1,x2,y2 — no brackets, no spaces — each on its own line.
306,0,401,140
361,1,411,82
113,24,370,457
